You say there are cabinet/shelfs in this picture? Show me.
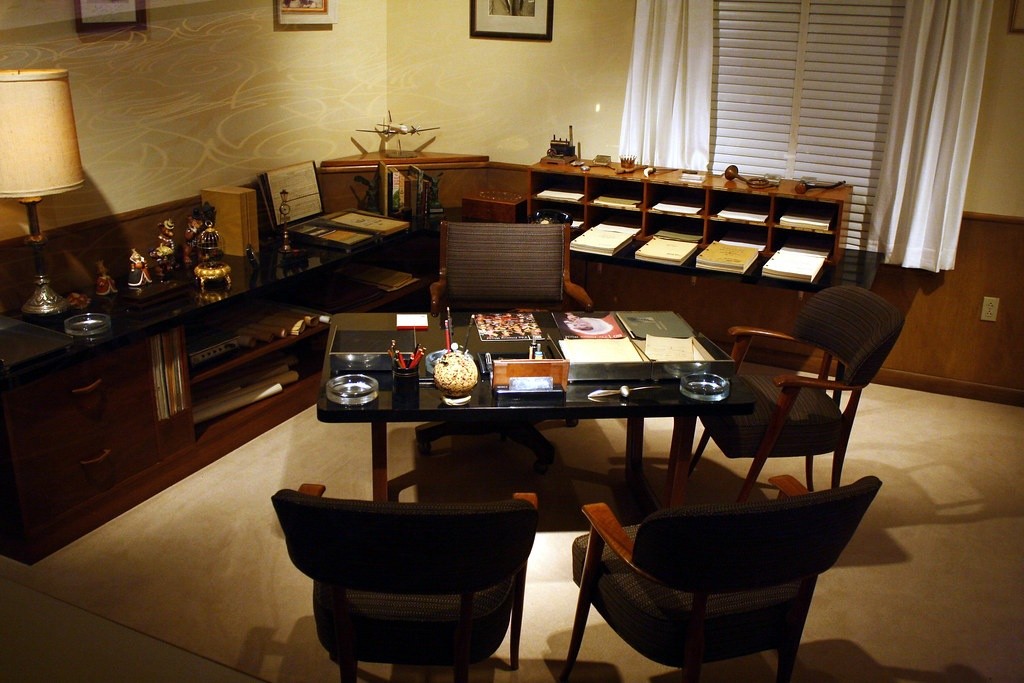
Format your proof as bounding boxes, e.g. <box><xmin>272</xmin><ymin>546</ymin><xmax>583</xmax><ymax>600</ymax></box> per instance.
<box><xmin>0</xmin><ymin>188</ymin><xmax>432</xmax><ymax>567</ymax></box>
<box><xmin>527</xmin><ymin>159</ymin><xmax>855</xmax><ymax>291</ymax></box>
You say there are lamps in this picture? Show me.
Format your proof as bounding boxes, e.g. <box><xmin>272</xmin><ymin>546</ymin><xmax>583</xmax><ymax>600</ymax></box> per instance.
<box><xmin>0</xmin><ymin>68</ymin><xmax>86</xmax><ymax>317</ymax></box>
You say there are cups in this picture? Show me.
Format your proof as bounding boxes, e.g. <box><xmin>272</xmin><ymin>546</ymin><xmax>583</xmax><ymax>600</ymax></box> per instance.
<box><xmin>391</xmin><ymin>358</ymin><xmax>420</xmax><ymax>405</ymax></box>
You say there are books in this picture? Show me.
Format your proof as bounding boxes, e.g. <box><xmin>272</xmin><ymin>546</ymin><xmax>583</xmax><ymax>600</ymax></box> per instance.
<box><xmin>388</xmin><ymin>165</ymin><xmax>430</xmax><ymax>215</ymax></box>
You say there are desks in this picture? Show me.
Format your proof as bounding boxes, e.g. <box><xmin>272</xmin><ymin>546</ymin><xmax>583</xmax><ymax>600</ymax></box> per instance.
<box><xmin>319</xmin><ymin>311</ymin><xmax>758</xmax><ymax>512</ymax></box>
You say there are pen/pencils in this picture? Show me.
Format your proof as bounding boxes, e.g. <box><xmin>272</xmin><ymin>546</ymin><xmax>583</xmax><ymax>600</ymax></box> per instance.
<box><xmin>463</xmin><ymin>314</ymin><xmax>475</xmax><ymax>355</ymax></box>
<box><xmin>445</xmin><ymin>306</ymin><xmax>453</xmax><ymax>353</ymax></box>
<box><xmin>477</xmin><ymin>353</ymin><xmax>487</xmax><ymax>374</ymax></box>
<box><xmin>387</xmin><ymin>338</ymin><xmax>427</xmax><ymax>370</ymax></box>
<box><xmin>531</xmin><ymin>336</ymin><xmax>544</xmax><ymax>360</ymax></box>
<box><xmin>486</xmin><ymin>352</ymin><xmax>492</xmax><ymax>385</ymax></box>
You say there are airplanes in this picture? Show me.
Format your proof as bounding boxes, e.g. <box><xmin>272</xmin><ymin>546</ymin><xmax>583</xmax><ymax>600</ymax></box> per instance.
<box><xmin>355</xmin><ymin>110</ymin><xmax>441</xmax><ymax>136</ymax></box>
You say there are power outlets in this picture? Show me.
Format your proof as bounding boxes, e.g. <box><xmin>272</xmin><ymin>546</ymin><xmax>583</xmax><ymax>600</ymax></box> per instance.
<box><xmin>980</xmin><ymin>297</ymin><xmax>999</xmax><ymax>322</ymax></box>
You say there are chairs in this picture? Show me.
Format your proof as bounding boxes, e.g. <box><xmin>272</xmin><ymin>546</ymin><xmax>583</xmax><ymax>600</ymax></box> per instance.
<box><xmin>270</xmin><ymin>220</ymin><xmax>906</xmax><ymax>682</ymax></box>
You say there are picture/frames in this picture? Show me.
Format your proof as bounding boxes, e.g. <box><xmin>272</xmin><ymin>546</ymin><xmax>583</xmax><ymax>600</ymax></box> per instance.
<box><xmin>469</xmin><ymin>0</ymin><xmax>554</xmax><ymax>41</ymax></box>
<box><xmin>75</xmin><ymin>0</ymin><xmax>147</xmax><ymax>33</ymax></box>
<box><xmin>276</xmin><ymin>0</ymin><xmax>339</xmax><ymax>25</ymax></box>
<box><xmin>1008</xmin><ymin>0</ymin><xmax>1024</xmax><ymax>35</ymax></box>
